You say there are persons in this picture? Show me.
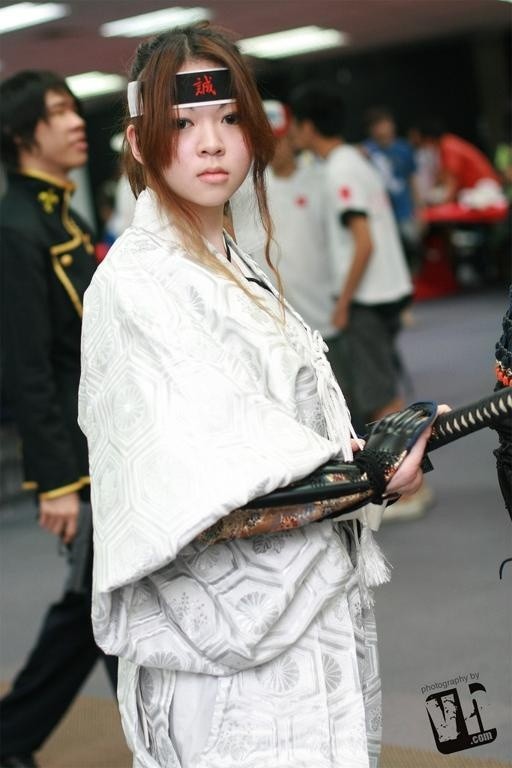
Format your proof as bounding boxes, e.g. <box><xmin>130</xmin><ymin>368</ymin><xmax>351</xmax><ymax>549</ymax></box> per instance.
<box><xmin>77</xmin><ymin>27</ymin><xmax>450</xmax><ymax>768</ymax></box>
<box><xmin>94</xmin><ymin>94</ymin><xmax>512</xmax><ymax>578</ymax></box>
<box><xmin>1</xmin><ymin>71</ymin><xmax>109</xmax><ymax>768</ymax></box>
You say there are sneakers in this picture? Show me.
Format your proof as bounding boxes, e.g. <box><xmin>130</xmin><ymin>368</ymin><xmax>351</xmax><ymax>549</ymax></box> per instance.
<box><xmin>378</xmin><ymin>479</ymin><xmax>437</xmax><ymax>521</ymax></box>
